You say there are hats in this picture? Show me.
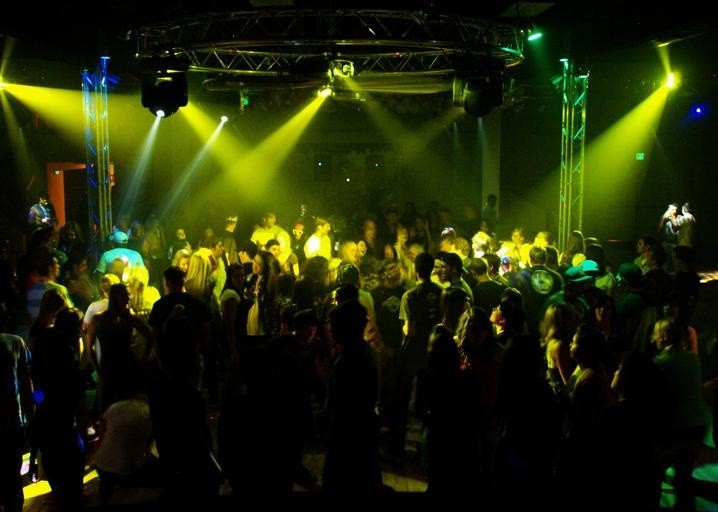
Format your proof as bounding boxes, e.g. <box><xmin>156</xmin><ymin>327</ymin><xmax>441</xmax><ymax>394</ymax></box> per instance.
<box><xmin>236</xmin><ymin>240</ymin><xmax>256</xmax><ymax>257</ymax></box>
<box><xmin>579</xmin><ymin>259</ymin><xmax>599</xmax><ymax>273</ymax></box>
<box><xmin>561</xmin><ymin>266</ymin><xmax>594</xmax><ymax>283</ymax></box>
<box><xmin>440</xmin><ymin>252</ymin><xmax>469</xmax><ymax>276</ymax></box>
<box><xmin>107</xmin><ymin>231</ymin><xmax>129</xmax><ymax>244</ymax></box>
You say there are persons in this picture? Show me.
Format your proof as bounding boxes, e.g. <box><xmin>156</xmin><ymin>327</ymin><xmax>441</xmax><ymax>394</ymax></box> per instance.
<box><xmin>2</xmin><ymin>193</ymin><xmax>717</xmax><ymax>511</ymax></box>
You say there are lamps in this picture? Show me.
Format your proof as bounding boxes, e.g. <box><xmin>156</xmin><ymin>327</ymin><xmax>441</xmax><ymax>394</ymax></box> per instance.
<box><xmin>286</xmin><ymin>56</ymin><xmax>369</xmax><ymax>106</ymax></box>
<box><xmin>137</xmin><ymin>39</ymin><xmax>187</xmax><ymax>121</ymax></box>
<box><xmin>451</xmin><ymin>54</ymin><xmax>505</xmax><ymax>120</ymax></box>
